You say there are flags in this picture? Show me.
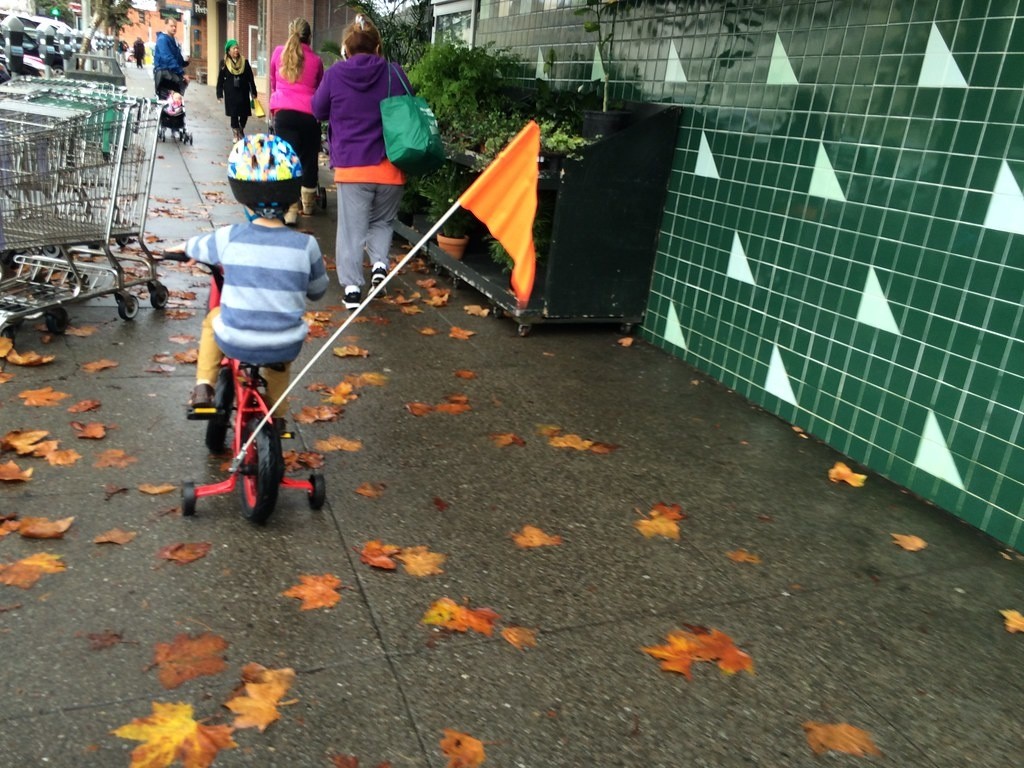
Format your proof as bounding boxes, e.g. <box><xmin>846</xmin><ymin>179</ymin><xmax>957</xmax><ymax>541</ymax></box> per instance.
<box><xmin>459</xmin><ymin>122</ymin><xmax>539</xmax><ymax>307</ymax></box>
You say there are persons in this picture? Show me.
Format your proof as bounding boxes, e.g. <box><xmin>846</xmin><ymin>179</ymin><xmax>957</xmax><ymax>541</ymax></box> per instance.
<box><xmin>162</xmin><ymin>133</ymin><xmax>328</xmax><ymax>433</ymax></box>
<box><xmin>313</xmin><ymin>14</ymin><xmax>414</xmax><ymax>311</ymax></box>
<box><xmin>216</xmin><ymin>39</ymin><xmax>257</xmax><ymax>144</ymax></box>
<box><xmin>268</xmin><ymin>18</ymin><xmax>324</xmax><ymax>225</ymax></box>
<box><xmin>153</xmin><ymin>18</ymin><xmax>191</xmax><ymax>131</ymax></box>
<box><xmin>133</xmin><ymin>36</ymin><xmax>145</xmax><ymax>68</ymax></box>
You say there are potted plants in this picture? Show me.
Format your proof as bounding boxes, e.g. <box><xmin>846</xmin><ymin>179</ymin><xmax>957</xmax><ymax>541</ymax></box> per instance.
<box><xmin>398</xmin><ymin>0</ymin><xmax>642</xmax><ymax>290</ymax></box>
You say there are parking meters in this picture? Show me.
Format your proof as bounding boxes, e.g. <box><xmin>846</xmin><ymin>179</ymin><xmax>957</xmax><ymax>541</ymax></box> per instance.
<box><xmin>0</xmin><ymin>17</ymin><xmax>114</xmax><ymax>73</ymax></box>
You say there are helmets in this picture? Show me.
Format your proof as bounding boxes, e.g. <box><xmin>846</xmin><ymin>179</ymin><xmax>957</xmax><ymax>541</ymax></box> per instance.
<box><xmin>227</xmin><ymin>133</ymin><xmax>303</xmax><ymax>221</ymax></box>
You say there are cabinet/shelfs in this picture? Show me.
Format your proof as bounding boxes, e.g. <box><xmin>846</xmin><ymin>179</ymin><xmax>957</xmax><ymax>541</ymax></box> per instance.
<box><xmin>394</xmin><ymin>91</ymin><xmax>684</xmax><ymax>338</ymax></box>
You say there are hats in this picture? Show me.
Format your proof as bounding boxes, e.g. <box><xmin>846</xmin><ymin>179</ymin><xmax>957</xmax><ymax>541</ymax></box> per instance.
<box><xmin>224</xmin><ymin>39</ymin><xmax>238</xmax><ymax>54</ymax></box>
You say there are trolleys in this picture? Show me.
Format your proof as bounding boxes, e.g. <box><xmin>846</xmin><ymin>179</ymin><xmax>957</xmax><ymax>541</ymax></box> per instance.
<box><xmin>0</xmin><ymin>73</ymin><xmax>172</xmax><ymax>334</ymax></box>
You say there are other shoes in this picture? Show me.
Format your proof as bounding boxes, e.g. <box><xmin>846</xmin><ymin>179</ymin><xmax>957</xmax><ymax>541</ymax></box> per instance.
<box><xmin>232</xmin><ymin>128</ymin><xmax>245</xmax><ymax>141</ymax></box>
<box><xmin>185</xmin><ymin>382</ymin><xmax>218</xmax><ymax>421</ymax></box>
<box><xmin>257</xmin><ymin>416</ymin><xmax>295</xmax><ymax>441</ymax></box>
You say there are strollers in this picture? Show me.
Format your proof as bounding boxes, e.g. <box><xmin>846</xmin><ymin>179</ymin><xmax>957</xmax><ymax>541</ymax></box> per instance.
<box><xmin>155</xmin><ymin>70</ymin><xmax>193</xmax><ymax>145</ymax></box>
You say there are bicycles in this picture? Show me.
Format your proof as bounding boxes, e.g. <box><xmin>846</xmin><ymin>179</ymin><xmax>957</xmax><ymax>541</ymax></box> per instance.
<box><xmin>162</xmin><ymin>250</ymin><xmax>326</xmax><ymax>526</ymax></box>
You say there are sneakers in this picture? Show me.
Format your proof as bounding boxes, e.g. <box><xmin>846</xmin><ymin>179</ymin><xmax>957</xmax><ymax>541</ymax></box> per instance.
<box><xmin>342</xmin><ymin>290</ymin><xmax>361</xmax><ymax>310</ymax></box>
<box><xmin>368</xmin><ymin>266</ymin><xmax>388</xmax><ymax>301</ymax></box>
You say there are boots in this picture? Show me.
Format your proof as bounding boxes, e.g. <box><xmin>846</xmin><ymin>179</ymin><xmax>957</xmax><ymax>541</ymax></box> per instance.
<box><xmin>284</xmin><ymin>202</ymin><xmax>299</xmax><ymax>224</ymax></box>
<box><xmin>301</xmin><ymin>186</ymin><xmax>319</xmax><ymax>218</ymax></box>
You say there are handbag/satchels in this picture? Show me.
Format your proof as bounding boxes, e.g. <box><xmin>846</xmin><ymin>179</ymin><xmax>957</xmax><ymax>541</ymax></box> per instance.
<box><xmin>250</xmin><ymin>97</ymin><xmax>266</xmax><ymax>118</ymax></box>
<box><xmin>379</xmin><ymin>61</ymin><xmax>443</xmax><ymax>163</ymax></box>
